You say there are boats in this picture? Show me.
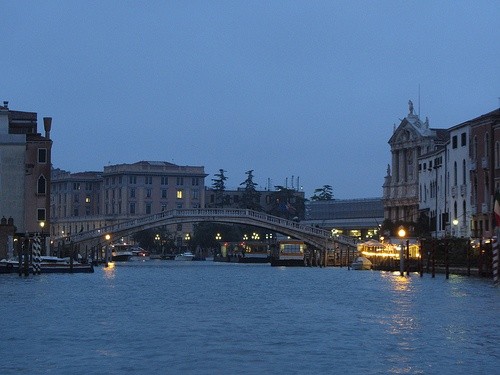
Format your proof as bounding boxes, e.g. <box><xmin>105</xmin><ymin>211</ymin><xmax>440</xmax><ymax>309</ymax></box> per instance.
<box><xmin>95</xmin><ymin>233</ymin><xmax>319</xmax><ymax>267</ymax></box>
<box><xmin>0</xmin><ymin>254</ymin><xmax>94</xmax><ymax>274</ymax></box>
<box><xmin>353</xmin><ymin>254</ymin><xmax>373</xmax><ymax>270</ymax></box>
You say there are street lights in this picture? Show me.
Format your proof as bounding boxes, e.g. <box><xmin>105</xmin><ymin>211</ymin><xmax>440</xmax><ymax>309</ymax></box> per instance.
<box><xmin>398</xmin><ymin>228</ymin><xmax>407</xmax><ymax>277</ymax></box>
<box><xmin>41</xmin><ymin>221</ymin><xmax>44</xmax><ymax>255</ymax></box>
